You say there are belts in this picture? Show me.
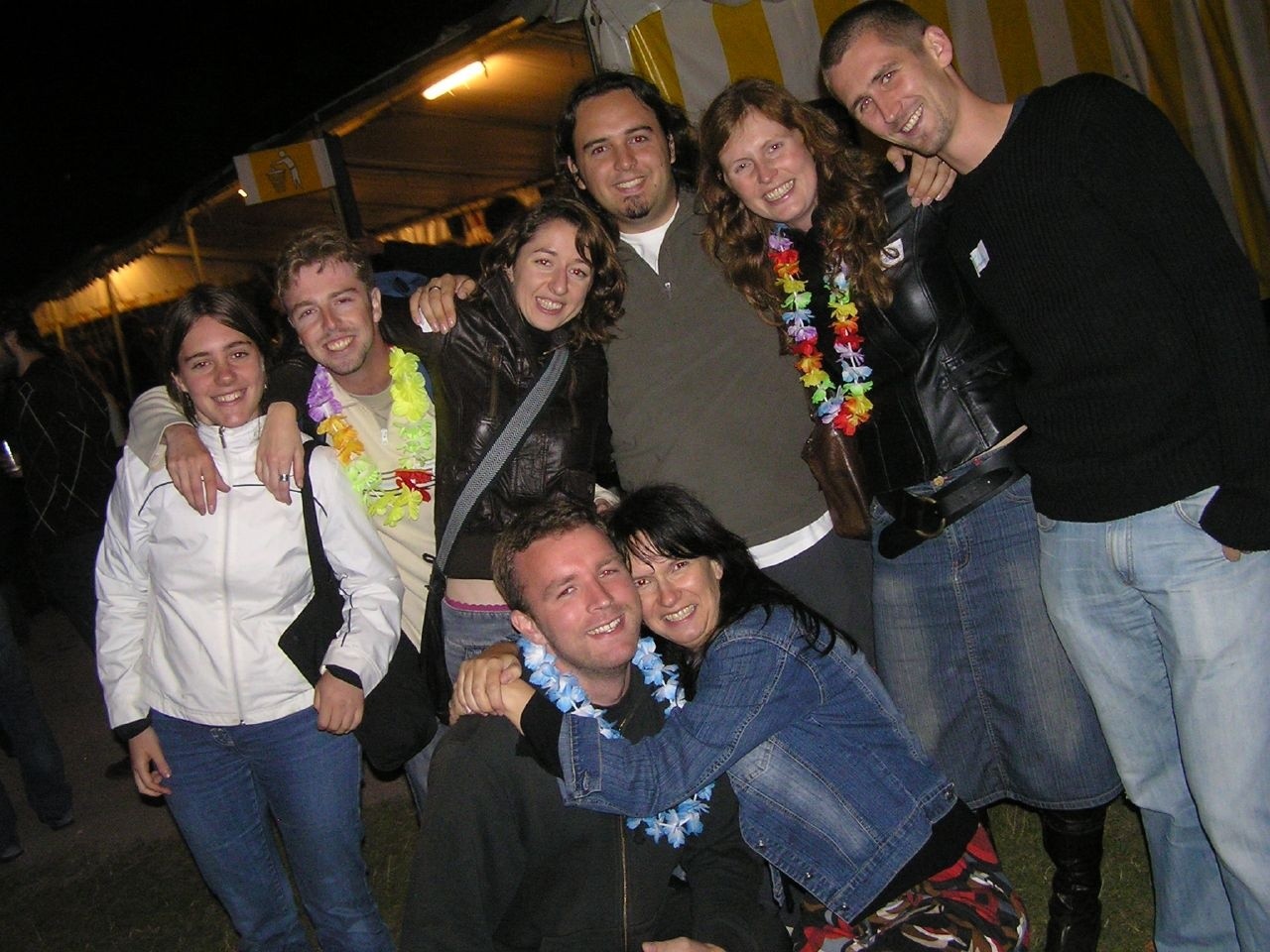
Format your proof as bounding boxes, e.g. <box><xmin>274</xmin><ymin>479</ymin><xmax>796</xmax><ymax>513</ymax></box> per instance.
<box><xmin>875</xmin><ymin>429</ymin><xmax>1033</xmax><ymax>560</ymax></box>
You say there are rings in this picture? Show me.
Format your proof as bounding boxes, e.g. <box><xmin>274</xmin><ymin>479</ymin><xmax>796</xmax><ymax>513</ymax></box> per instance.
<box><xmin>938</xmin><ymin>160</ymin><xmax>946</xmax><ymax>164</ymax></box>
<box><xmin>428</xmin><ymin>286</ymin><xmax>441</xmax><ymax>292</ymax></box>
<box><xmin>279</xmin><ymin>474</ymin><xmax>288</xmax><ymax>481</ymax></box>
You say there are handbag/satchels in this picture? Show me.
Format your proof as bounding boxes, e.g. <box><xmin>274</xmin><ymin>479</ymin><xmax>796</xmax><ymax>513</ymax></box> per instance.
<box><xmin>278</xmin><ymin>583</ymin><xmax>438</xmax><ymax>772</ymax></box>
<box><xmin>421</xmin><ymin>554</ymin><xmax>454</xmax><ymax>726</ymax></box>
<box><xmin>802</xmin><ymin>413</ymin><xmax>872</xmax><ymax>540</ymax></box>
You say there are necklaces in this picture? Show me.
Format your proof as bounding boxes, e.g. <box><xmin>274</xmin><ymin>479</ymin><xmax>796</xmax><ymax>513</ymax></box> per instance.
<box><xmin>305</xmin><ymin>347</ymin><xmax>436</xmax><ymax>532</ymax></box>
<box><xmin>515</xmin><ymin>635</ymin><xmax>716</xmax><ymax>848</ymax></box>
<box><xmin>765</xmin><ymin>227</ymin><xmax>874</xmax><ymax>437</ymax></box>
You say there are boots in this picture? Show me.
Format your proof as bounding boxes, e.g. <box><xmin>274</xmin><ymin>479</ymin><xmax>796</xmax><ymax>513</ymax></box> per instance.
<box><xmin>1039</xmin><ymin>809</ymin><xmax>1105</xmax><ymax>952</ymax></box>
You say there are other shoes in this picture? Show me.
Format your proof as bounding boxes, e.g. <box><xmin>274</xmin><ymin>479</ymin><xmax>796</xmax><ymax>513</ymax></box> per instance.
<box><xmin>50</xmin><ymin>808</ymin><xmax>73</xmax><ymax>831</ymax></box>
<box><xmin>0</xmin><ymin>844</ymin><xmax>24</xmax><ymax>862</ymax></box>
<box><xmin>105</xmin><ymin>756</ymin><xmax>134</xmax><ymax>777</ymax></box>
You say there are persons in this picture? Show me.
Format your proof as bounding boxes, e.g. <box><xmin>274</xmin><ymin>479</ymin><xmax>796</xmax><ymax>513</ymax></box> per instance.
<box><xmin>409</xmin><ymin>49</ymin><xmax>956</xmax><ymax>683</ymax></box>
<box><xmin>692</xmin><ymin>72</ymin><xmax>1120</xmax><ymax>952</ymax></box>
<box><xmin>816</xmin><ymin>0</ymin><xmax>1270</xmax><ymax>952</ymax></box>
<box><xmin>1</xmin><ymin>302</ymin><xmax>127</xmax><ymax>860</ymax></box>
<box><xmin>256</xmin><ymin>196</ymin><xmax>628</xmax><ymax>825</ymax></box>
<box><xmin>449</xmin><ymin>484</ymin><xmax>1032</xmax><ymax>952</ymax></box>
<box><xmin>397</xmin><ymin>487</ymin><xmax>812</xmax><ymax>952</ymax></box>
<box><xmin>92</xmin><ymin>230</ymin><xmax>446</xmax><ymax>952</ymax></box>
